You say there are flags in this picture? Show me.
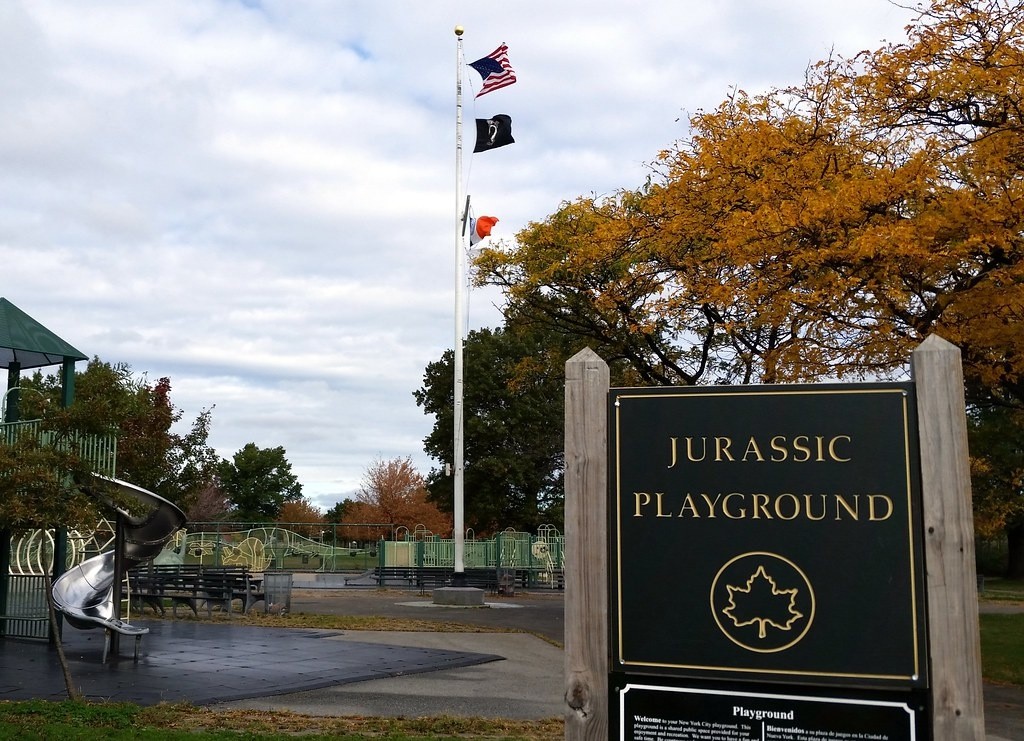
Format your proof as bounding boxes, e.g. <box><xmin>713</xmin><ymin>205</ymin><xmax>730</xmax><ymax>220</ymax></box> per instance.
<box><xmin>473</xmin><ymin>114</ymin><xmax>515</xmax><ymax>153</ymax></box>
<box><xmin>470</xmin><ymin>216</ymin><xmax>500</xmax><ymax>246</ymax></box>
<box><xmin>467</xmin><ymin>42</ymin><xmax>516</xmax><ymax>98</ymax></box>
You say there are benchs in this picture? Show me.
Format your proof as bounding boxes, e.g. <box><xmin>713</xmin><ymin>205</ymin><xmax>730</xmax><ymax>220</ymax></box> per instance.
<box><xmin>0</xmin><ymin>575</ymin><xmax>52</xmax><ymax>639</ymax></box>
<box><xmin>371</xmin><ymin>567</ymin><xmax>529</xmax><ymax>597</ymax></box>
<box><xmin>121</xmin><ymin>564</ymin><xmax>266</xmax><ymax>621</ymax></box>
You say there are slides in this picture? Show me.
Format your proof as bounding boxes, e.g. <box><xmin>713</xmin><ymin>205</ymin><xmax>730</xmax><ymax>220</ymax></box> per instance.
<box><xmin>48</xmin><ymin>463</ymin><xmax>189</xmax><ymax>635</ymax></box>
